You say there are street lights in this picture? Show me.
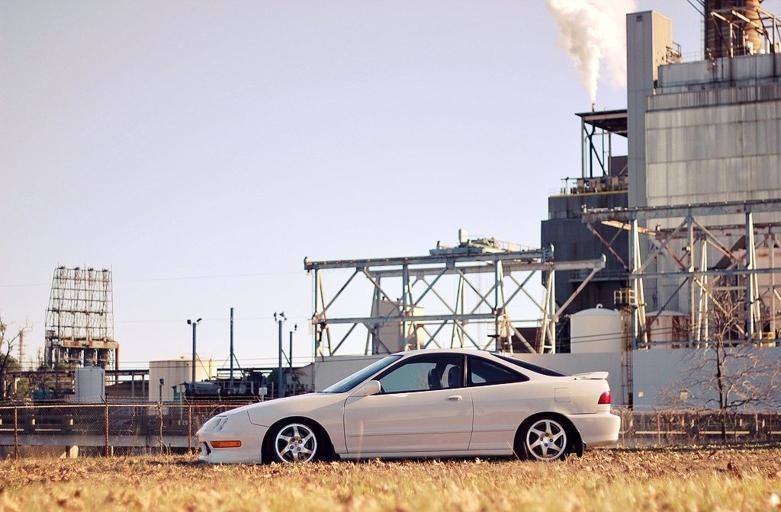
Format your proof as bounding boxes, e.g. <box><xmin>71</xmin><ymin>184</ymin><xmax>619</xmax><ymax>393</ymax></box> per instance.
<box><xmin>159</xmin><ymin>377</ymin><xmax>165</xmax><ymax>404</ymax></box>
<box><xmin>273</xmin><ymin>312</ymin><xmax>287</xmax><ymax>397</ymax></box>
<box><xmin>290</xmin><ymin>323</ymin><xmax>299</xmax><ymax>391</ymax></box>
<box><xmin>186</xmin><ymin>318</ymin><xmax>204</xmax><ymax>401</ymax></box>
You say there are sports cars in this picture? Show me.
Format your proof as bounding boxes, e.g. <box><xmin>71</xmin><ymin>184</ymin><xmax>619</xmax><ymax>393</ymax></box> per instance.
<box><xmin>194</xmin><ymin>349</ymin><xmax>621</xmax><ymax>468</ymax></box>
